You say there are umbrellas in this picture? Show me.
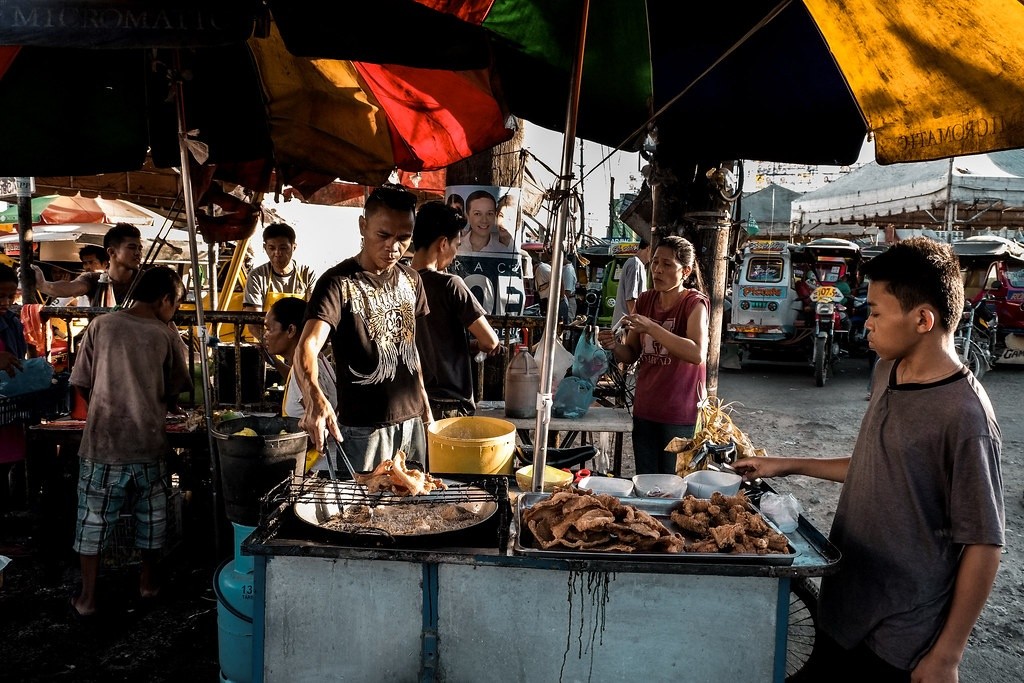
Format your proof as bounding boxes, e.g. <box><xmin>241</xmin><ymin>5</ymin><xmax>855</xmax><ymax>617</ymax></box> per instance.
<box><xmin>419</xmin><ymin>0</ymin><xmax>1024</xmax><ymax>510</ymax></box>
<box><xmin>0</xmin><ymin>0</ymin><xmax>517</xmax><ymax>522</ymax></box>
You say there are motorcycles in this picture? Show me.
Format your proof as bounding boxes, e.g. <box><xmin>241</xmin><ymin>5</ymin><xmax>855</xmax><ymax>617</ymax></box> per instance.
<box><xmin>595</xmin><ymin>254</ymin><xmax>636</xmax><ymax>330</ymax></box>
<box><xmin>798</xmin><ymin>238</ymin><xmax>866</xmax><ymax>385</ymax></box>
<box><xmin>950</xmin><ymin>235</ymin><xmax>1024</xmax><ymax>380</ymax></box>
<box><xmin>729</xmin><ymin>240</ymin><xmax>803</xmax><ymax>349</ymax></box>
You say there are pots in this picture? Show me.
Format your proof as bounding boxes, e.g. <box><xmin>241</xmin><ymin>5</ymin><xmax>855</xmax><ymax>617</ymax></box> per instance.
<box><xmin>293</xmin><ymin>478</ymin><xmax>499</xmax><ymax>545</ymax></box>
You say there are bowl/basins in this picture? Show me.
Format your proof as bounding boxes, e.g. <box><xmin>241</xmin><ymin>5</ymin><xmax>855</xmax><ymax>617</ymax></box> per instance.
<box><xmin>515</xmin><ymin>463</ymin><xmax>574</xmax><ymax>492</ymax></box>
<box><xmin>632</xmin><ymin>474</ymin><xmax>687</xmax><ymax>498</ymax></box>
<box><xmin>684</xmin><ymin>469</ymin><xmax>742</xmax><ymax>498</ymax></box>
<box><xmin>578</xmin><ymin>476</ymin><xmax>633</xmax><ymax>497</ymax></box>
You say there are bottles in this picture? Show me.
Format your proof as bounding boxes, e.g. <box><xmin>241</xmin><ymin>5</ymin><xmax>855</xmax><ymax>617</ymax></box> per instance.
<box><xmin>504</xmin><ymin>346</ymin><xmax>539</xmax><ymax>417</ymax></box>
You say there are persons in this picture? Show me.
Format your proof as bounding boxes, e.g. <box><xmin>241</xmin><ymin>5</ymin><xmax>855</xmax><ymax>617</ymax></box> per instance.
<box><xmin>292</xmin><ymin>182</ymin><xmax>438</xmax><ymax>475</ymax></box>
<box><xmin>262</xmin><ymin>296</ymin><xmax>339</xmax><ymax>480</ymax></box>
<box><xmin>241</xmin><ymin>222</ymin><xmax>322</xmax><ymax>397</ymax></box>
<box><xmin>491</xmin><ymin>195</ymin><xmax>521</xmax><ymax>253</ymax></box>
<box><xmin>727</xmin><ymin>236</ymin><xmax>1007</xmax><ymax>683</ymax></box>
<box><xmin>0</xmin><ymin>221</ymin><xmax>194</xmax><ymax>618</ymax></box>
<box><xmin>406</xmin><ymin>199</ymin><xmax>500</xmax><ymax>421</ymax></box>
<box><xmin>608</xmin><ymin>238</ymin><xmax>650</xmax><ymax>390</ymax></box>
<box><xmin>534</xmin><ymin>247</ymin><xmax>578</xmax><ymax>330</ymax></box>
<box><xmin>445</xmin><ymin>193</ymin><xmax>465</xmax><ymax>216</ymax></box>
<box><xmin>458</xmin><ymin>190</ymin><xmax>507</xmax><ymax>252</ymax></box>
<box><xmin>596</xmin><ymin>234</ymin><xmax>711</xmax><ymax>476</ymax></box>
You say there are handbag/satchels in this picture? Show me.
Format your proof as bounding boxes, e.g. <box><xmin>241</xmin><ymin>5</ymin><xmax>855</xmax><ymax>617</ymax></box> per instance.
<box><xmin>551</xmin><ymin>375</ymin><xmax>594</xmax><ymax>418</ymax></box>
<box><xmin>572</xmin><ymin>330</ymin><xmax>610</xmax><ymax>380</ymax></box>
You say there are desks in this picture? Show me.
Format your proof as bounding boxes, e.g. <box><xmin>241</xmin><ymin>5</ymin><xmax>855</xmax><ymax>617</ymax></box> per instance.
<box><xmin>28</xmin><ymin>405</ymin><xmax>635</xmax><ymax>481</ymax></box>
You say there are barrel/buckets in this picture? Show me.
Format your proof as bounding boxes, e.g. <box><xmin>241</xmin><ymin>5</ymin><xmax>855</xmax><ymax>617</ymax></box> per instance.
<box><xmin>427</xmin><ymin>416</ymin><xmax>516</xmax><ymax>474</ymax></box>
<box><xmin>211</xmin><ymin>416</ymin><xmax>309</xmax><ymax>525</ymax></box>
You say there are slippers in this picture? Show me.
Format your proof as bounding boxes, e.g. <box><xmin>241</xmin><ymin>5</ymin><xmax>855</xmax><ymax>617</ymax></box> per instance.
<box><xmin>59</xmin><ymin>595</ymin><xmax>95</xmax><ymax>621</ymax></box>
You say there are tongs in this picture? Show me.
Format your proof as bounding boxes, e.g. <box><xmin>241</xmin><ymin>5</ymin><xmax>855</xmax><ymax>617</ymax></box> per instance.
<box><xmin>323</xmin><ymin>427</ymin><xmax>368</xmax><ymax>515</ymax></box>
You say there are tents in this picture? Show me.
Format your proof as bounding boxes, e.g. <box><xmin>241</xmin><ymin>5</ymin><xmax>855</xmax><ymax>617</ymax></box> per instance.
<box><xmin>727</xmin><ymin>145</ymin><xmax>1024</xmax><ymax>237</ymax></box>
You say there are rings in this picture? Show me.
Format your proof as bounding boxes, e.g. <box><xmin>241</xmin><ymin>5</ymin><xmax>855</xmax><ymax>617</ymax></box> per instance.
<box><xmin>600</xmin><ymin>340</ymin><xmax>603</xmax><ymax>344</ymax></box>
